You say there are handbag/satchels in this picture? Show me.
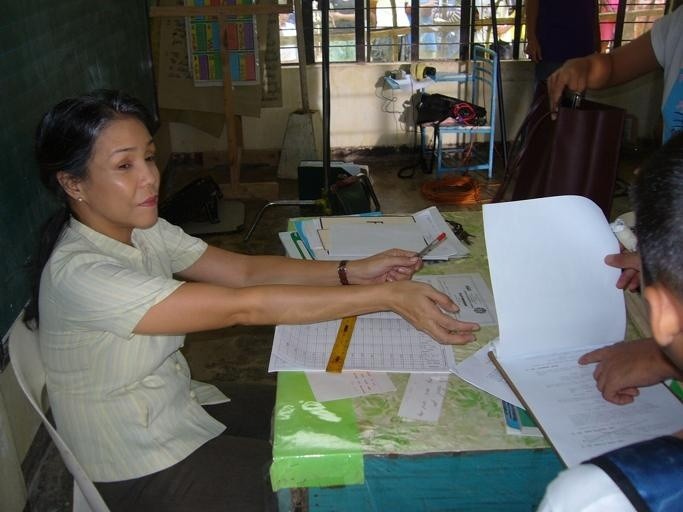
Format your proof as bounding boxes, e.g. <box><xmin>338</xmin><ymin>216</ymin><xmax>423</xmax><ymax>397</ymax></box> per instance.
<box><xmin>492</xmin><ymin>80</ymin><xmax>626</xmax><ymax>221</ymax></box>
<box><xmin>412</xmin><ymin>91</ymin><xmax>486</xmax><ymax>126</ymax></box>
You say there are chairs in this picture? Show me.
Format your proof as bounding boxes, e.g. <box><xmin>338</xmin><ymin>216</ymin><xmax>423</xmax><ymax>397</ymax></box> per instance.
<box><xmin>6</xmin><ymin>303</ymin><xmax>111</xmax><ymax>511</ymax></box>
<box><xmin>419</xmin><ymin>44</ymin><xmax>499</xmax><ymax>181</ymax></box>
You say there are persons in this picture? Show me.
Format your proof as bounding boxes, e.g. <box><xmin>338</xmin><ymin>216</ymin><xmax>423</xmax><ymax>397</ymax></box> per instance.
<box><xmin>524</xmin><ymin>1</ymin><xmax>600</xmax><ymax>106</ymax></box>
<box><xmin>537</xmin><ymin>126</ymin><xmax>683</xmax><ymax>512</ymax></box>
<box><xmin>22</xmin><ymin>88</ymin><xmax>482</xmax><ymax>512</ymax></box>
<box><xmin>547</xmin><ymin>5</ymin><xmax>683</xmax><ymax>146</ymax></box>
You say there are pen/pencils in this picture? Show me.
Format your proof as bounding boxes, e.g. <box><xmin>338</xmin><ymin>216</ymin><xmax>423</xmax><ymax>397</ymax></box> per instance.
<box><xmin>416</xmin><ymin>233</ymin><xmax>445</xmax><ymax>257</ymax></box>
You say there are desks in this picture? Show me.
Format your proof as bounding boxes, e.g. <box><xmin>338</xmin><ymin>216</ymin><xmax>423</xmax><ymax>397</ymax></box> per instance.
<box><xmin>262</xmin><ymin>206</ymin><xmax>567</xmax><ymax>511</ymax></box>
<box><xmin>383</xmin><ymin>72</ymin><xmax>476</xmax><ymax>97</ymax></box>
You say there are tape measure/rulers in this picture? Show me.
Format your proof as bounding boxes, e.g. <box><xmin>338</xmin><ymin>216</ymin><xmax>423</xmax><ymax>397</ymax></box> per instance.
<box><xmin>326</xmin><ymin>317</ymin><xmax>356</xmax><ymax>372</ymax></box>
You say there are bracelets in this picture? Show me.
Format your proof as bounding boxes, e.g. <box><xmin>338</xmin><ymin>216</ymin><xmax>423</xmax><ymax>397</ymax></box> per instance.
<box><xmin>336</xmin><ymin>258</ymin><xmax>351</xmax><ymax>287</ymax></box>
